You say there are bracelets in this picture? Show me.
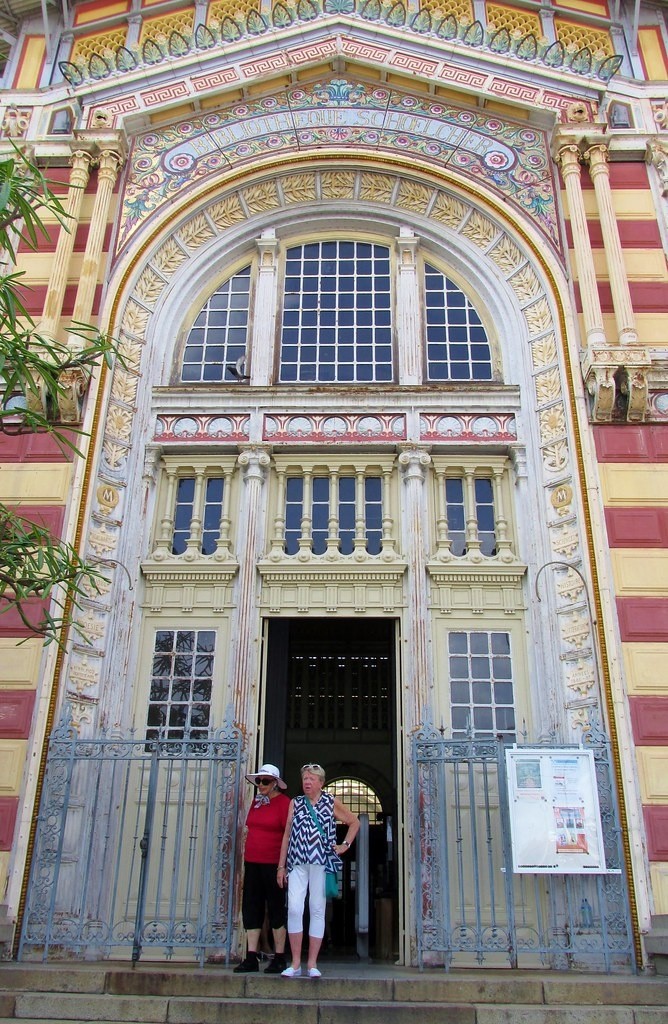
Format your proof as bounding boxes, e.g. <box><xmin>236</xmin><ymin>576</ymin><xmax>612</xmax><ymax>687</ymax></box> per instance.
<box><xmin>341</xmin><ymin>840</ymin><xmax>352</xmax><ymax>849</ymax></box>
<box><xmin>276</xmin><ymin>865</ymin><xmax>285</xmax><ymax>871</ymax></box>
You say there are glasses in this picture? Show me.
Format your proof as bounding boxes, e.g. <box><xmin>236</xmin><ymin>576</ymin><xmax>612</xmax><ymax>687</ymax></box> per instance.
<box><xmin>303</xmin><ymin>765</ymin><xmax>322</xmax><ymax>770</ymax></box>
<box><xmin>255</xmin><ymin>777</ymin><xmax>275</xmax><ymax>786</ymax></box>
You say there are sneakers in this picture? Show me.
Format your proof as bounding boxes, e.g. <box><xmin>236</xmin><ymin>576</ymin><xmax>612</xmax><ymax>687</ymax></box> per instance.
<box><xmin>307</xmin><ymin>968</ymin><xmax>321</xmax><ymax>977</ymax></box>
<box><xmin>264</xmin><ymin>958</ymin><xmax>286</xmax><ymax>973</ymax></box>
<box><xmin>281</xmin><ymin>966</ymin><xmax>301</xmax><ymax>976</ymax></box>
<box><xmin>234</xmin><ymin>957</ymin><xmax>259</xmax><ymax>972</ymax></box>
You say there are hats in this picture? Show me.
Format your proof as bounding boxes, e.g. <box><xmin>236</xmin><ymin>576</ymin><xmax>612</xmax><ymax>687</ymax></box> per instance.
<box><xmin>245</xmin><ymin>764</ymin><xmax>287</xmax><ymax>789</ymax></box>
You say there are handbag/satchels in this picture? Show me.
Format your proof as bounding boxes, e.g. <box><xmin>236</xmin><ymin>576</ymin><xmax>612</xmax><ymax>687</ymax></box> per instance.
<box><xmin>326</xmin><ymin>872</ymin><xmax>339</xmax><ymax>897</ymax></box>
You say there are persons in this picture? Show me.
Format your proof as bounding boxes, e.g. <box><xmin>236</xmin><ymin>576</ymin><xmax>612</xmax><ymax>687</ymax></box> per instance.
<box><xmin>233</xmin><ymin>764</ymin><xmax>291</xmax><ymax>973</ymax></box>
<box><xmin>276</xmin><ymin>765</ymin><xmax>360</xmax><ymax>977</ymax></box>
